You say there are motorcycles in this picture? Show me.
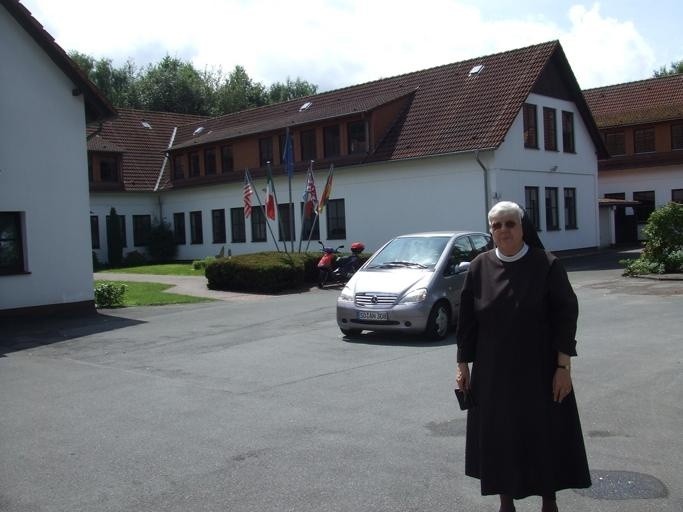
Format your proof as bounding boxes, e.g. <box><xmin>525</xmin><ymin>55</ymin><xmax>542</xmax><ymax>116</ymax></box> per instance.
<box><xmin>316</xmin><ymin>240</ymin><xmax>363</xmax><ymax>288</ymax></box>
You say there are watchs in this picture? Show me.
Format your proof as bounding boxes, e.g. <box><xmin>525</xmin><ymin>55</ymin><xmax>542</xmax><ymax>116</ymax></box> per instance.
<box><xmin>556</xmin><ymin>364</ymin><xmax>570</xmax><ymax>371</ymax></box>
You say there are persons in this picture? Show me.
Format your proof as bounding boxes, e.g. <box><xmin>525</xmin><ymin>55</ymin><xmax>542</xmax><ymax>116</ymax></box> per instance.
<box><xmin>454</xmin><ymin>201</ymin><xmax>591</xmax><ymax>512</ymax></box>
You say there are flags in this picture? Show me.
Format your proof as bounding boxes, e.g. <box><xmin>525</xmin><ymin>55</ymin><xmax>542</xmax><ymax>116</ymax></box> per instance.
<box><xmin>241</xmin><ymin>128</ymin><xmax>335</xmax><ymax>222</ymax></box>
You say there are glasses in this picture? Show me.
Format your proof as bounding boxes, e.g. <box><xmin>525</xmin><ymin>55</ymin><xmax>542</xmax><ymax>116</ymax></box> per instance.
<box><xmin>492</xmin><ymin>220</ymin><xmax>516</xmax><ymax>230</ymax></box>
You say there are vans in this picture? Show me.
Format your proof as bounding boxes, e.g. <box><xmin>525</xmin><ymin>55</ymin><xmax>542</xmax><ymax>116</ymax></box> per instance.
<box><xmin>337</xmin><ymin>232</ymin><xmax>491</xmax><ymax>342</ymax></box>
